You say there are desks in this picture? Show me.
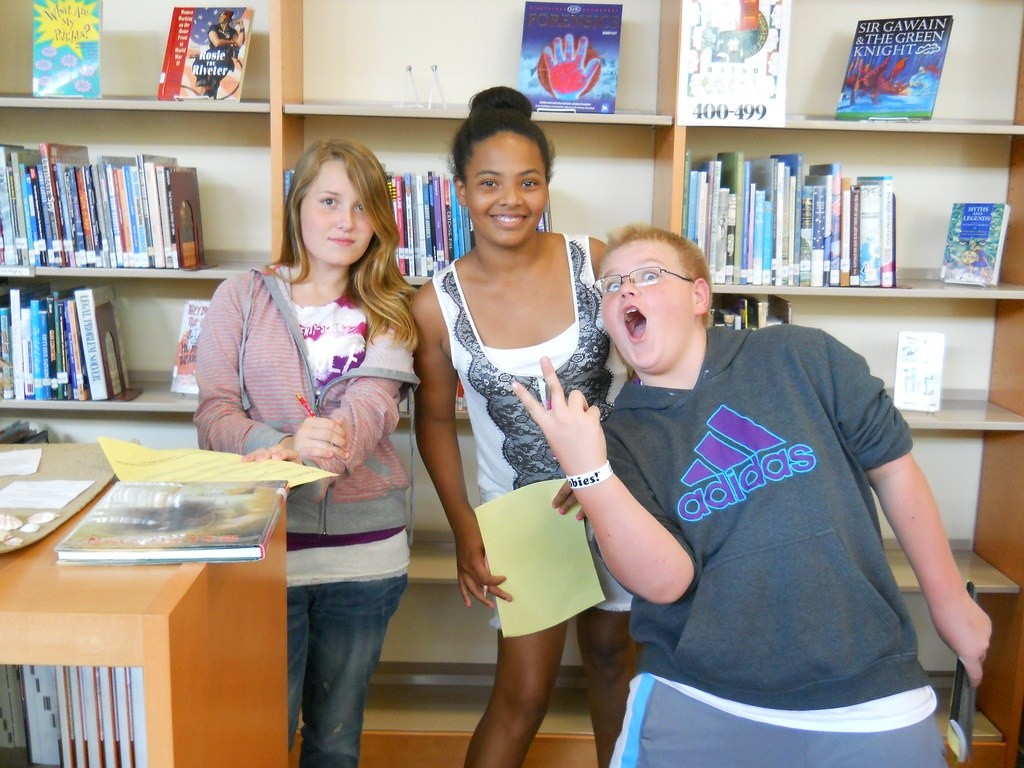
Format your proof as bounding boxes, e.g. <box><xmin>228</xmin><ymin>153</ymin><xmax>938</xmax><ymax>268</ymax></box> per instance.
<box><xmin>0</xmin><ymin>483</ymin><xmax>290</xmax><ymax>768</ymax></box>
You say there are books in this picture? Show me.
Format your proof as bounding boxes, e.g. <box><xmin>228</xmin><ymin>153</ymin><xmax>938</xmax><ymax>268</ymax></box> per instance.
<box><xmin>284</xmin><ymin>170</ymin><xmax>296</xmax><ymax>199</ymax></box>
<box><xmin>383</xmin><ymin>172</ymin><xmax>476</xmax><ymax>278</ymax></box>
<box><xmin>941</xmin><ymin>203</ymin><xmax>1011</xmax><ymax>286</ymax></box>
<box><xmin>535</xmin><ymin>193</ymin><xmax>552</xmax><ymax>233</ymax></box>
<box><xmin>710</xmin><ymin>295</ymin><xmax>792</xmax><ymax>331</ymax></box>
<box><xmin>0</xmin><ymin>142</ymin><xmax>204</xmax><ymax>269</ymax></box>
<box><xmin>680</xmin><ymin>148</ymin><xmax>897</xmax><ymax>289</ymax></box>
<box><xmin>0</xmin><ymin>283</ymin><xmax>130</xmax><ymax>401</ymax></box>
<box><xmin>170</xmin><ymin>299</ymin><xmax>211</xmax><ymax>395</ymax></box>
<box><xmin>56</xmin><ymin>479</ymin><xmax>288</xmax><ymax>563</ymax></box>
<box><xmin>834</xmin><ymin>15</ymin><xmax>954</xmax><ymax>119</ymax></box>
<box><xmin>32</xmin><ymin>0</ymin><xmax>103</xmax><ymax>99</ymax></box>
<box><xmin>932</xmin><ymin>687</ymin><xmax>1002</xmax><ymax>742</ymax></box>
<box><xmin>158</xmin><ymin>6</ymin><xmax>254</xmax><ymax>102</ymax></box>
<box><xmin>518</xmin><ymin>1</ymin><xmax>623</xmax><ymax>114</ymax></box>
<box><xmin>893</xmin><ymin>331</ymin><xmax>945</xmax><ymax>413</ymax></box>
<box><xmin>0</xmin><ymin>420</ymin><xmax>49</xmax><ymax>444</ymax></box>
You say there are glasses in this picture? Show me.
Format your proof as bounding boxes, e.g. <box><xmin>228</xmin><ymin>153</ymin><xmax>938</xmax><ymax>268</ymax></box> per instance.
<box><xmin>593</xmin><ymin>265</ymin><xmax>694</xmax><ymax>297</ymax></box>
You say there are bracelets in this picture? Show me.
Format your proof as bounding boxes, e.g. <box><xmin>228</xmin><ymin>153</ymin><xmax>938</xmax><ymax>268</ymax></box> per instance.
<box><xmin>567</xmin><ymin>461</ymin><xmax>613</xmax><ymax>489</ymax></box>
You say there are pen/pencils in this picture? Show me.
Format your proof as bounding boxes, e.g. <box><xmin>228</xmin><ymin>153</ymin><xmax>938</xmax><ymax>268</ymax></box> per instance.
<box><xmin>294</xmin><ymin>392</ymin><xmax>316</xmax><ymax>419</ymax></box>
<box><xmin>482</xmin><ymin>552</ymin><xmax>489</xmax><ymax>599</ymax></box>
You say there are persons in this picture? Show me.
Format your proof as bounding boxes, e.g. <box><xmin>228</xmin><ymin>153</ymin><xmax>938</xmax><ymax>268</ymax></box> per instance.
<box><xmin>408</xmin><ymin>88</ymin><xmax>639</xmax><ymax>768</ymax></box>
<box><xmin>513</xmin><ymin>225</ymin><xmax>993</xmax><ymax>768</ymax></box>
<box><xmin>193</xmin><ymin>138</ymin><xmax>421</xmax><ymax>768</ymax></box>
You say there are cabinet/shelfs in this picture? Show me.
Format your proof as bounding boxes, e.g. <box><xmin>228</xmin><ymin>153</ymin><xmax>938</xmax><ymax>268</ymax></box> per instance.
<box><xmin>1</xmin><ymin>0</ymin><xmax>1022</xmax><ymax>767</ymax></box>
